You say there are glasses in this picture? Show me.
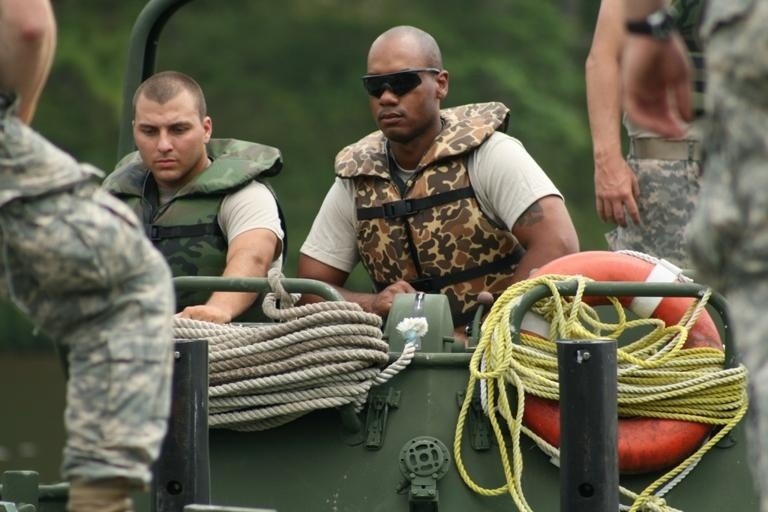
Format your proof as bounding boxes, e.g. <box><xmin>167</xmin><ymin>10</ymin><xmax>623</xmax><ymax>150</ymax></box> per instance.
<box><xmin>360</xmin><ymin>68</ymin><xmax>440</xmax><ymax>99</ymax></box>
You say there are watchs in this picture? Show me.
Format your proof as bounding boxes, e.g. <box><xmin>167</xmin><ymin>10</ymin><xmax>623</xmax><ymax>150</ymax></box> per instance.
<box><xmin>626</xmin><ymin>10</ymin><xmax>676</xmax><ymax>39</ymax></box>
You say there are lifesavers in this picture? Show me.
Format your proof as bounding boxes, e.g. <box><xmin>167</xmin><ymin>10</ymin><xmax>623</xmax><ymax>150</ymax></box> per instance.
<box><xmin>495</xmin><ymin>250</ymin><xmax>723</xmax><ymax>471</ymax></box>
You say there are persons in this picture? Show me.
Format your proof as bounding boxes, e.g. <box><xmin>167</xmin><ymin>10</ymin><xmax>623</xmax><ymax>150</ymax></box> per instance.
<box><xmin>586</xmin><ymin>0</ymin><xmax>708</xmax><ymax>271</ymax></box>
<box><xmin>101</xmin><ymin>71</ymin><xmax>287</xmax><ymax>325</ymax></box>
<box><xmin>294</xmin><ymin>25</ymin><xmax>580</xmax><ymax>347</ymax></box>
<box><xmin>0</xmin><ymin>0</ymin><xmax>176</xmax><ymax>512</ymax></box>
<box><xmin>621</xmin><ymin>0</ymin><xmax>768</xmax><ymax>512</ymax></box>
